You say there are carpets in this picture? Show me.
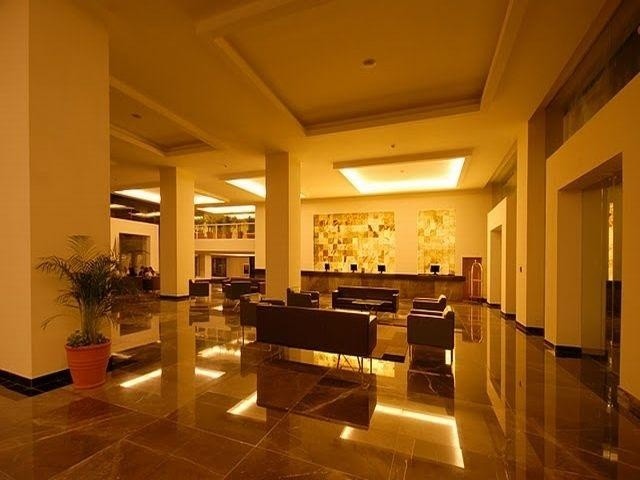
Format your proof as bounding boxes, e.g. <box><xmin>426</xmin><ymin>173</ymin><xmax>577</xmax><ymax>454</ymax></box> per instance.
<box><xmin>366</xmin><ymin>322</ymin><xmax>409</xmax><ymax>364</ymax></box>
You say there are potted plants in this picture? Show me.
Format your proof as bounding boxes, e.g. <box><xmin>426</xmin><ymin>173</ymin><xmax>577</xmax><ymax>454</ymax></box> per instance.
<box><xmin>32</xmin><ymin>232</ymin><xmax>142</xmax><ymax>389</ymax></box>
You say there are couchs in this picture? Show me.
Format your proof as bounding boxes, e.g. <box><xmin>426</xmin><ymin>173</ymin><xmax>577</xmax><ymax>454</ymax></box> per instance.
<box><xmin>331</xmin><ymin>286</ymin><xmax>399</xmax><ymax>319</ymax></box>
<box><xmin>256</xmin><ymin>302</ymin><xmax>377</xmax><ymax>376</ymax></box>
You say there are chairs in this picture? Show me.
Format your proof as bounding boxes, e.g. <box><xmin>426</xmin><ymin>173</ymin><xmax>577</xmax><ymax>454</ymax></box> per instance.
<box><xmin>287</xmin><ymin>288</ymin><xmax>320</xmax><ymax>308</ymax></box>
<box><xmin>124</xmin><ymin>274</ymin><xmax>285</xmax><ymax>339</ymax></box>
<box><xmin>406</xmin><ymin>304</ymin><xmax>455</xmax><ymax>364</ymax></box>
<box><xmin>412</xmin><ymin>293</ymin><xmax>447</xmax><ymax>311</ymax></box>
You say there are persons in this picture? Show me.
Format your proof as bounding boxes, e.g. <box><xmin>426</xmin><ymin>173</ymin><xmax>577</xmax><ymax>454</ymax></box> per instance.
<box><xmin>127</xmin><ymin>266</ymin><xmax>156</xmax><ymax>280</ymax></box>
